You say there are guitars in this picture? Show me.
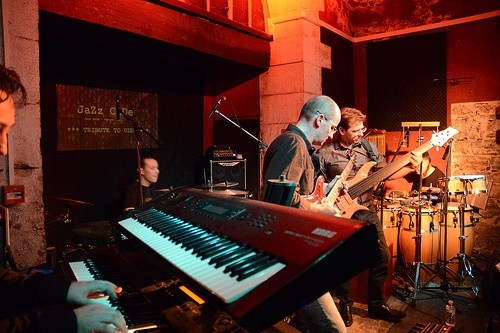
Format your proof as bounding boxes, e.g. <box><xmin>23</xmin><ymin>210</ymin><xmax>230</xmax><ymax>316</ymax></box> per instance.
<box><xmin>334</xmin><ymin>125</ymin><xmax>462</xmax><ymax>219</ymax></box>
<box><xmin>300</xmin><ymin>151</ymin><xmax>356</xmax><ymax>206</ymax></box>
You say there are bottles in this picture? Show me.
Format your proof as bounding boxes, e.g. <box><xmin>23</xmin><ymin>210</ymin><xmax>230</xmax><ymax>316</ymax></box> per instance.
<box><xmin>445</xmin><ymin>301</ymin><xmax>456</xmax><ymax>327</ymax></box>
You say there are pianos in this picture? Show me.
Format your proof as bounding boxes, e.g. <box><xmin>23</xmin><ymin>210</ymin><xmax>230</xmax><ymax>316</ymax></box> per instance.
<box><xmin>56</xmin><ymin>186</ymin><xmax>382</xmax><ymax>333</ymax></box>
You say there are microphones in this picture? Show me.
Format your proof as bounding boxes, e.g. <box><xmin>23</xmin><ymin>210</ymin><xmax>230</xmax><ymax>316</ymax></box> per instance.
<box><xmin>208</xmin><ymin>99</ymin><xmax>222</xmax><ymax>119</ymax></box>
<box><xmin>407</xmin><ymin>127</ymin><xmax>409</xmax><ymax>147</ymax></box>
<box><xmin>116</xmin><ymin>95</ymin><xmax>120</xmax><ymax>120</ymax></box>
<box><xmin>442</xmin><ymin>144</ymin><xmax>449</xmax><ymax>160</ymax></box>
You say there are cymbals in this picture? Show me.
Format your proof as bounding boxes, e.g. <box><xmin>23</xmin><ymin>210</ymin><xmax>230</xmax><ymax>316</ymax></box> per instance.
<box><xmin>156</xmin><ymin>189</ymin><xmax>248</xmax><ymax>196</ymax></box>
<box><xmin>214</xmin><ymin>182</ymin><xmax>239</xmax><ymax>187</ymax></box>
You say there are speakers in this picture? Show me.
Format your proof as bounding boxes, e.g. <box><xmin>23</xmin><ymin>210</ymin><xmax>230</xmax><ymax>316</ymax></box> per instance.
<box><xmin>205</xmin><ymin>158</ymin><xmax>247</xmax><ymax>198</ymax></box>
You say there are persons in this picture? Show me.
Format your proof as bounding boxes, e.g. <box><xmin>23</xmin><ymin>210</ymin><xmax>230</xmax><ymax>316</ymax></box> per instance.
<box><xmin>317</xmin><ymin>108</ymin><xmax>422</xmax><ymax>327</ymax></box>
<box><xmin>262</xmin><ymin>94</ymin><xmax>350</xmax><ymax>333</ymax></box>
<box><xmin>124</xmin><ymin>155</ymin><xmax>159</xmax><ymax>211</ymax></box>
<box><xmin>405</xmin><ymin>150</ymin><xmax>445</xmax><ymax>193</ymax></box>
<box><xmin>0</xmin><ymin>65</ymin><xmax>127</xmax><ymax>333</ymax></box>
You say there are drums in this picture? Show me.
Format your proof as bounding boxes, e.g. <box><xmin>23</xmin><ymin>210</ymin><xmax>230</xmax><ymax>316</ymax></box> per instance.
<box><xmin>373</xmin><ymin>202</ymin><xmax>401</xmax><ymax>272</ymax></box>
<box><xmin>399</xmin><ymin>206</ymin><xmax>440</xmax><ymax>285</ymax></box>
<box><xmin>436</xmin><ymin>202</ymin><xmax>474</xmax><ymax>282</ymax></box>
<box><xmin>439</xmin><ymin>174</ymin><xmax>487</xmax><ymax>196</ymax></box>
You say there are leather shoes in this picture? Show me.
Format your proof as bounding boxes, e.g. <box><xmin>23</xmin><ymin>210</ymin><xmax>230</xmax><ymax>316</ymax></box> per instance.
<box><xmin>368</xmin><ymin>305</ymin><xmax>405</xmax><ymax>321</ymax></box>
<box><xmin>340</xmin><ymin>305</ymin><xmax>353</xmax><ymax>325</ymax></box>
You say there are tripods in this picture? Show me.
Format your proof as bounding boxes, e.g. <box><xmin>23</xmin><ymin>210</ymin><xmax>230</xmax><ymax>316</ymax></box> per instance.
<box><xmin>392</xmin><ymin>127</ymin><xmax>484</xmax><ymax>308</ymax></box>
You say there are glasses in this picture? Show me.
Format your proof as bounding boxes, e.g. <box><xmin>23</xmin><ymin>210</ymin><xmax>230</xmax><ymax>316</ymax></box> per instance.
<box><xmin>317</xmin><ymin>110</ymin><xmax>338</xmax><ymax>135</ymax></box>
<box><xmin>345</xmin><ymin>128</ymin><xmax>367</xmax><ymax>135</ymax></box>
